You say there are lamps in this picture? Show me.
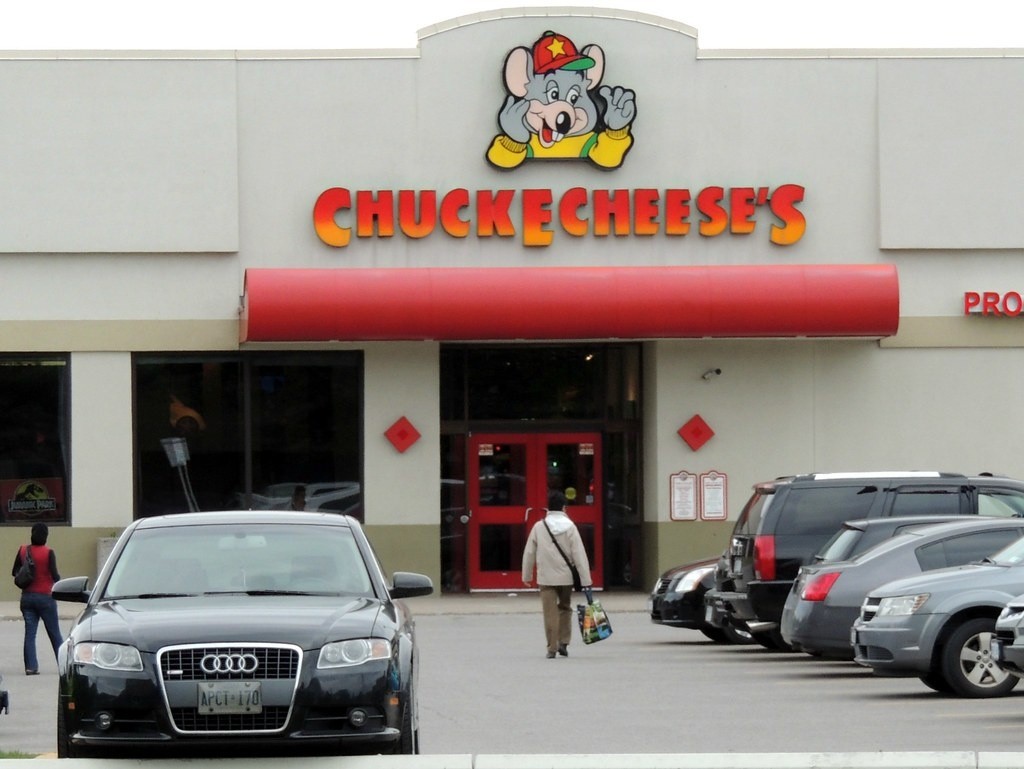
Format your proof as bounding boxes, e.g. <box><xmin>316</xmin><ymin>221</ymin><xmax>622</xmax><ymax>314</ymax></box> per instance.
<box><xmin>702</xmin><ymin>368</ymin><xmax>721</xmax><ymax>380</ymax></box>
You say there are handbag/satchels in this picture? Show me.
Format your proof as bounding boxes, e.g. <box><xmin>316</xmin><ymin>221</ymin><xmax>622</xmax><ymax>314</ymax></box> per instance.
<box><xmin>572</xmin><ymin>566</ymin><xmax>582</xmax><ymax>593</ymax></box>
<box><xmin>14</xmin><ymin>546</ymin><xmax>35</xmax><ymax>590</ymax></box>
<box><xmin>576</xmin><ymin>586</ymin><xmax>612</xmax><ymax>644</ymax></box>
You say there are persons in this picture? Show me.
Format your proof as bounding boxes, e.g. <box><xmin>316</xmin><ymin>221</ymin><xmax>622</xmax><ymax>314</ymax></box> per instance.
<box><xmin>12</xmin><ymin>523</ymin><xmax>63</xmax><ymax>675</ymax></box>
<box><xmin>522</xmin><ymin>495</ymin><xmax>592</xmax><ymax>658</ymax></box>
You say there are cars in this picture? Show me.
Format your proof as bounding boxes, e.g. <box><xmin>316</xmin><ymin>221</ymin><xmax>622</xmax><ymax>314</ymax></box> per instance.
<box><xmin>227</xmin><ymin>482</ymin><xmax>641</xmax><ymax>587</ymax></box>
<box><xmin>780</xmin><ymin>515</ymin><xmax>1024</xmax><ymax>698</ymax></box>
<box><xmin>52</xmin><ymin>507</ymin><xmax>433</xmax><ymax>759</ymax></box>
<box><xmin>652</xmin><ymin>550</ymin><xmax>727</xmax><ymax>642</ymax></box>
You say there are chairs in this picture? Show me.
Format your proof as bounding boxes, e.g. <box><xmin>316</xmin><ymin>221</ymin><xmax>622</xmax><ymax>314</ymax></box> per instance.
<box><xmin>160</xmin><ymin>559</ymin><xmax>203</xmax><ymax>587</ymax></box>
<box><xmin>291</xmin><ymin>556</ymin><xmax>334</xmax><ymax>581</ymax></box>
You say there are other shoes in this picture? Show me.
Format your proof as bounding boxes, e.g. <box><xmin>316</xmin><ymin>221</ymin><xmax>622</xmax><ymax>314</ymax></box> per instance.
<box><xmin>546</xmin><ymin>651</ymin><xmax>556</xmax><ymax>658</ymax></box>
<box><xmin>558</xmin><ymin>644</ymin><xmax>568</xmax><ymax>657</ymax></box>
<box><xmin>26</xmin><ymin>668</ymin><xmax>40</xmax><ymax>676</ymax></box>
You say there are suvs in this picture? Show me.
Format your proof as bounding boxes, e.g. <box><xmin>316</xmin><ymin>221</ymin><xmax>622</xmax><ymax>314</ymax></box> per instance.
<box><xmin>700</xmin><ymin>469</ymin><xmax>1024</xmax><ymax>649</ymax></box>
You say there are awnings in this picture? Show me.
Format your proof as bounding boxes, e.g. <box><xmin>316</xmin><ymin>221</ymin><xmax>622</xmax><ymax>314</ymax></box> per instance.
<box><xmin>238</xmin><ymin>264</ymin><xmax>899</xmax><ymax>339</ymax></box>
